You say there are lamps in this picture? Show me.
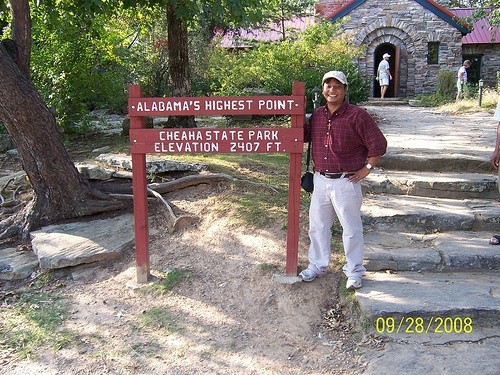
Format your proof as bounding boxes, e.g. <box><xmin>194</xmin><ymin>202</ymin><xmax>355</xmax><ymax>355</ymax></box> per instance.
<box><xmin>311</xmin><ymin>86</ymin><xmax>321</xmax><ymax>102</ymax></box>
<box><xmin>479</xmin><ymin>78</ymin><xmax>484</xmax><ymax>86</ymax></box>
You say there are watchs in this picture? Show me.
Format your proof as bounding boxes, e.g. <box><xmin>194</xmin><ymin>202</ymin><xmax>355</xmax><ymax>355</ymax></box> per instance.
<box><xmin>365</xmin><ymin>164</ymin><xmax>373</xmax><ymax>169</ymax></box>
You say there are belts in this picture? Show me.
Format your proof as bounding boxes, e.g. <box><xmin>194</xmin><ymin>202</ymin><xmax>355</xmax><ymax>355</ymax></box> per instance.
<box><xmin>320</xmin><ymin>172</ymin><xmax>354</xmax><ymax>179</ymax></box>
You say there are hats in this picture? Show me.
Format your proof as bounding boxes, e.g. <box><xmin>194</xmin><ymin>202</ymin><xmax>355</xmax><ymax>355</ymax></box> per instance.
<box><xmin>383</xmin><ymin>53</ymin><xmax>391</xmax><ymax>59</ymax></box>
<box><xmin>322</xmin><ymin>71</ymin><xmax>348</xmax><ymax>91</ymax></box>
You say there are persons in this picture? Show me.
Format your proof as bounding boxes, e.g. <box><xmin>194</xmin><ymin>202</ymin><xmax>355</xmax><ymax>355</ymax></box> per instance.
<box><xmin>456</xmin><ymin>60</ymin><xmax>471</xmax><ymax>99</ymax></box>
<box><xmin>489</xmin><ymin>102</ymin><xmax>500</xmax><ymax>244</ymax></box>
<box><xmin>298</xmin><ymin>71</ymin><xmax>388</xmax><ymax>290</ymax></box>
<box><xmin>376</xmin><ymin>53</ymin><xmax>392</xmax><ymax>98</ymax></box>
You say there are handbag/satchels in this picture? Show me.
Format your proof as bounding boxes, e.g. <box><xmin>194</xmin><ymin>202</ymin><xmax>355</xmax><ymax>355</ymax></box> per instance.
<box><xmin>300</xmin><ymin>172</ymin><xmax>313</xmax><ymax>193</ymax></box>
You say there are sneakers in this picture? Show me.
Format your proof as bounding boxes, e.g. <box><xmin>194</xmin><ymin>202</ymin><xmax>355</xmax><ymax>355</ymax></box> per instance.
<box><xmin>346</xmin><ymin>276</ymin><xmax>362</xmax><ymax>290</ymax></box>
<box><xmin>298</xmin><ymin>268</ymin><xmax>327</xmax><ymax>282</ymax></box>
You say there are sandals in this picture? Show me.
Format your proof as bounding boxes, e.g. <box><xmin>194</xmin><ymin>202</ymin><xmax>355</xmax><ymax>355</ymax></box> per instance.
<box><xmin>489</xmin><ymin>234</ymin><xmax>500</xmax><ymax>245</ymax></box>
